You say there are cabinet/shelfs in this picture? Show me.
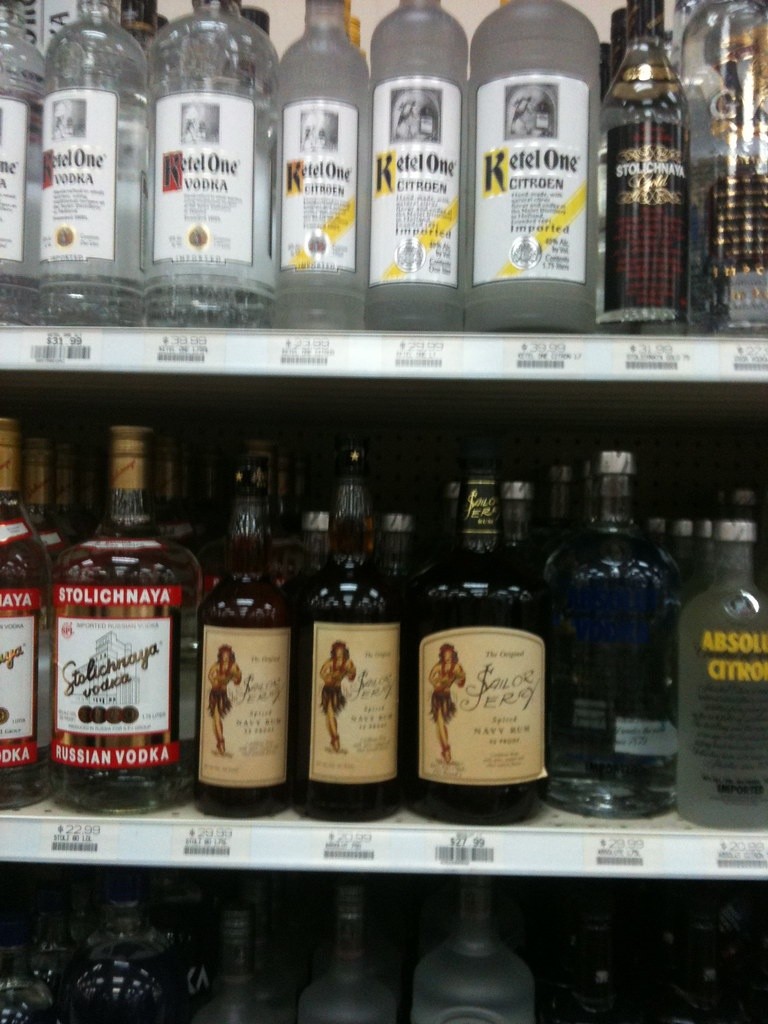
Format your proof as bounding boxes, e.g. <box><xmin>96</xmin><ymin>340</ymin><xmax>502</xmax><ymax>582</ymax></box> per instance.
<box><xmin>0</xmin><ymin>322</ymin><xmax>768</xmax><ymax>875</ymax></box>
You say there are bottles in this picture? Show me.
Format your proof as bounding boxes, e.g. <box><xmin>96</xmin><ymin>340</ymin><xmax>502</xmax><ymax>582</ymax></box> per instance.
<box><xmin>1</xmin><ymin>863</ymin><xmax>768</xmax><ymax>1024</ymax></box>
<box><xmin>0</xmin><ymin>415</ymin><xmax>768</xmax><ymax>828</ymax></box>
<box><xmin>0</xmin><ymin>0</ymin><xmax>768</xmax><ymax>339</ymax></box>
